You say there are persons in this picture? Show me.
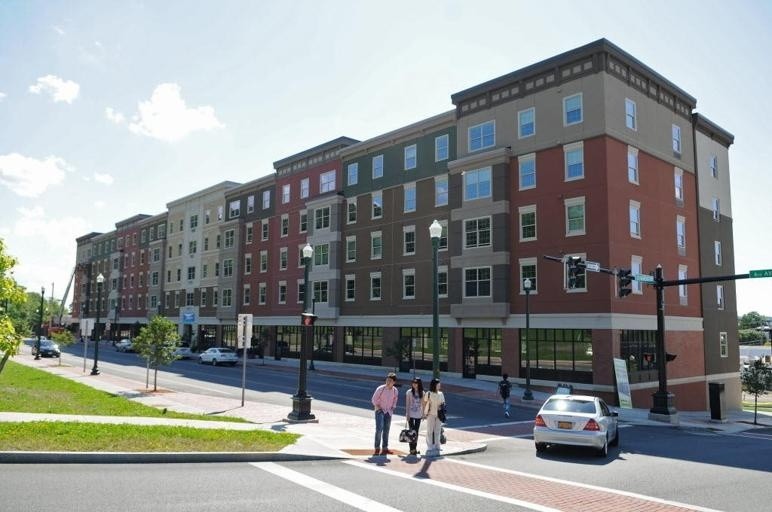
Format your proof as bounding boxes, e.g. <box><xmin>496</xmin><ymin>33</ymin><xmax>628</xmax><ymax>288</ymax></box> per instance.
<box><xmin>404</xmin><ymin>377</ymin><xmax>426</xmax><ymax>455</ymax></box>
<box><xmin>421</xmin><ymin>378</ymin><xmax>447</xmax><ymax>453</ymax></box>
<box><xmin>496</xmin><ymin>373</ymin><xmax>513</xmax><ymax>419</ymax></box>
<box><xmin>371</xmin><ymin>371</ymin><xmax>399</xmax><ymax>456</ymax></box>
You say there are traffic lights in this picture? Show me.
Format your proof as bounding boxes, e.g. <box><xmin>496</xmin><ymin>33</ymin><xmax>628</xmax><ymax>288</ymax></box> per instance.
<box><xmin>301</xmin><ymin>314</ymin><xmax>316</xmax><ymax>327</ymax></box>
<box><xmin>564</xmin><ymin>254</ymin><xmax>587</xmax><ymax>288</ymax></box>
<box><xmin>667</xmin><ymin>353</ymin><xmax>677</xmax><ymax>362</ymax></box>
<box><xmin>617</xmin><ymin>267</ymin><xmax>635</xmax><ymax>298</ymax></box>
<box><xmin>643</xmin><ymin>354</ymin><xmax>654</xmax><ymax>361</ymax></box>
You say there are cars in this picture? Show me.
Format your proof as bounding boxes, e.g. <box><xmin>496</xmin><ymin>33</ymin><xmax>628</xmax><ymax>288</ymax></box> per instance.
<box><xmin>530</xmin><ymin>391</ymin><xmax>620</xmax><ymax>458</ymax></box>
<box><xmin>198</xmin><ymin>347</ymin><xmax>240</xmax><ymax>367</ymax></box>
<box><xmin>586</xmin><ymin>347</ymin><xmax>593</xmax><ymax>356</ymax></box>
<box><xmin>113</xmin><ymin>338</ymin><xmax>138</xmax><ymax>353</ymax></box>
<box><xmin>31</xmin><ymin>338</ymin><xmax>61</xmax><ymax>358</ymax></box>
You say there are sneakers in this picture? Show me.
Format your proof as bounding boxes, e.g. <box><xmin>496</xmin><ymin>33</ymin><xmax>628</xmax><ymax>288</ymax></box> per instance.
<box><xmin>409</xmin><ymin>448</ymin><xmax>443</xmax><ymax>455</ymax></box>
<box><xmin>374</xmin><ymin>449</ymin><xmax>380</xmax><ymax>455</ymax></box>
<box><xmin>382</xmin><ymin>449</ymin><xmax>394</xmax><ymax>455</ymax></box>
<box><xmin>503</xmin><ymin>412</ymin><xmax>510</xmax><ymax>419</ymax></box>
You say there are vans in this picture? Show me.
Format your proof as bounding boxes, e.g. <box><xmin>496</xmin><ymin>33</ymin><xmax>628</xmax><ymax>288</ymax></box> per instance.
<box><xmin>255</xmin><ymin>341</ymin><xmax>289</xmax><ymax>356</ymax></box>
<box><xmin>172</xmin><ymin>341</ymin><xmax>192</xmax><ymax>359</ymax></box>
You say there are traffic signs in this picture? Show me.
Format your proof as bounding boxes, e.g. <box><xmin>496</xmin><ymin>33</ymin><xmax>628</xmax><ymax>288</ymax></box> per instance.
<box><xmin>749</xmin><ymin>269</ymin><xmax>772</xmax><ymax>278</ymax></box>
<box><xmin>635</xmin><ymin>273</ymin><xmax>654</xmax><ymax>282</ymax></box>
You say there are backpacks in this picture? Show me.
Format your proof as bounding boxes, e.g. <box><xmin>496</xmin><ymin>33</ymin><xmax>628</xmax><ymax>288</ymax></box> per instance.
<box><xmin>499</xmin><ymin>381</ymin><xmax>512</xmax><ymax>398</ymax></box>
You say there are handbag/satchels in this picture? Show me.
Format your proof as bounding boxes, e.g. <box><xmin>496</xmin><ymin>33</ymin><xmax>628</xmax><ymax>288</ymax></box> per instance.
<box><xmin>399</xmin><ymin>429</ymin><xmax>419</xmax><ymax>443</ymax></box>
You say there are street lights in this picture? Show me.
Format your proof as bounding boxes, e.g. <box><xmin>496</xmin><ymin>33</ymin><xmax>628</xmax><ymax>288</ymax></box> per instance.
<box><xmin>34</xmin><ymin>284</ymin><xmax>46</xmax><ymax>360</ymax></box>
<box><xmin>308</xmin><ymin>293</ymin><xmax>318</xmax><ymax>371</ymax></box>
<box><xmin>521</xmin><ymin>277</ymin><xmax>535</xmax><ymax>401</ymax></box>
<box><xmin>112</xmin><ymin>302</ymin><xmax>120</xmax><ymax>340</ymax></box>
<box><xmin>157</xmin><ymin>301</ymin><xmax>162</xmax><ymax>315</ymax></box>
<box><xmin>89</xmin><ymin>272</ymin><xmax>106</xmax><ymax>377</ymax></box>
<box><xmin>280</xmin><ymin>240</ymin><xmax>319</xmax><ymax>424</ymax></box>
<box><xmin>427</xmin><ymin>218</ymin><xmax>444</xmax><ymax>381</ymax></box>
